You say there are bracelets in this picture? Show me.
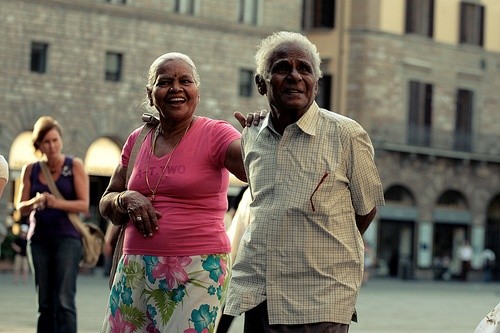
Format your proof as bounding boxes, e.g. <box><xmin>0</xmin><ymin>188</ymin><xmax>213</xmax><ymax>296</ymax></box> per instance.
<box><xmin>119</xmin><ymin>191</ymin><xmax>126</xmax><ymax>212</ymax></box>
<box><xmin>115</xmin><ymin>194</ymin><xmax>124</xmax><ymax>215</ymax></box>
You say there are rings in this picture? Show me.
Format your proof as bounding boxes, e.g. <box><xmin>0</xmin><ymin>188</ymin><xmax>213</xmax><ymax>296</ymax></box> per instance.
<box><xmin>136</xmin><ymin>217</ymin><xmax>141</xmax><ymax>221</ymax></box>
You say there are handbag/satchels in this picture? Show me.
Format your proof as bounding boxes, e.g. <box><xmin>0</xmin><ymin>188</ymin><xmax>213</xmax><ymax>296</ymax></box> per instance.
<box><xmin>78</xmin><ymin>222</ymin><xmax>105</xmax><ymax>269</ymax></box>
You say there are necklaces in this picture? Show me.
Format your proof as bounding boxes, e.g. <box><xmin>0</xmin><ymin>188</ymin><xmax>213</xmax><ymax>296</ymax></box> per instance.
<box><xmin>46</xmin><ymin>161</ymin><xmax>58</xmax><ymax>174</ymax></box>
<box><xmin>145</xmin><ymin>116</ymin><xmax>191</xmax><ymax>200</ymax></box>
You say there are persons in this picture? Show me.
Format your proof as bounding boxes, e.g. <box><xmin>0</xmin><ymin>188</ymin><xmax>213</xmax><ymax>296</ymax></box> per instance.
<box><xmin>434</xmin><ymin>255</ymin><xmax>450</xmax><ymax>279</ymax></box>
<box><xmin>98</xmin><ymin>52</ymin><xmax>267</xmax><ymax>333</ymax></box>
<box><xmin>361</xmin><ymin>241</ymin><xmax>375</xmax><ymax>285</ymax></box>
<box><xmin>17</xmin><ymin>116</ymin><xmax>89</xmax><ymax>333</ymax></box>
<box><xmin>0</xmin><ymin>154</ymin><xmax>9</xmax><ymax>200</ymax></box>
<box><xmin>459</xmin><ymin>240</ymin><xmax>473</xmax><ymax>281</ymax></box>
<box><xmin>224</xmin><ymin>31</ymin><xmax>385</xmax><ymax>333</ymax></box>
<box><xmin>1</xmin><ymin>201</ymin><xmax>121</xmax><ymax>274</ymax></box>
